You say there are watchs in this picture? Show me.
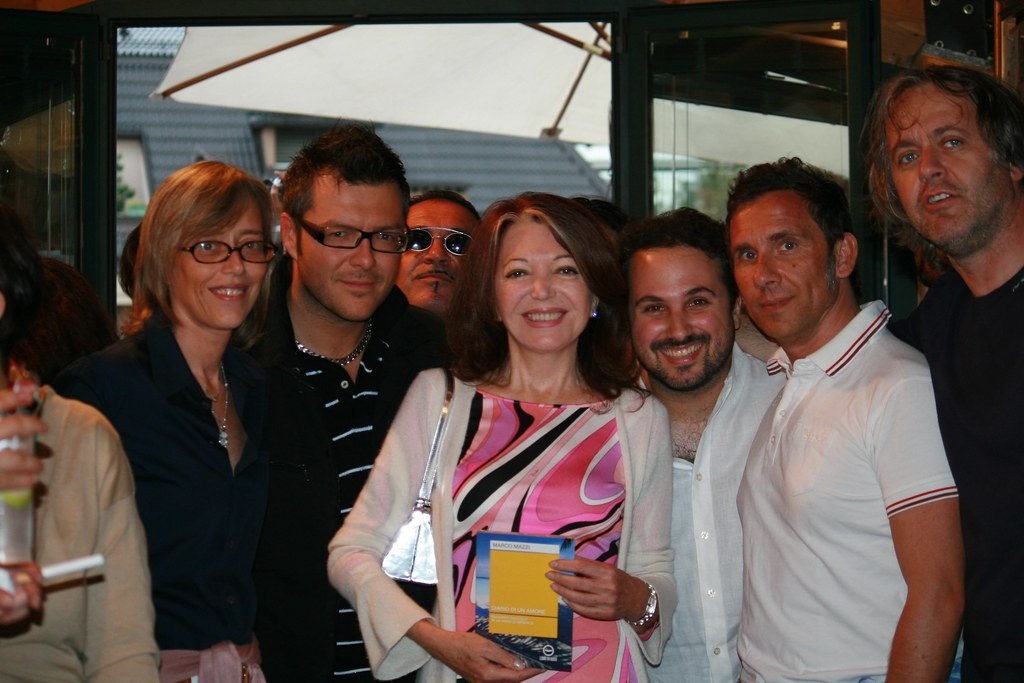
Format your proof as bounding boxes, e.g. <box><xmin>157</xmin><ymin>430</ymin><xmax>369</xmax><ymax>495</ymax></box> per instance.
<box><xmin>624</xmin><ymin>578</ymin><xmax>659</xmax><ymax>626</ymax></box>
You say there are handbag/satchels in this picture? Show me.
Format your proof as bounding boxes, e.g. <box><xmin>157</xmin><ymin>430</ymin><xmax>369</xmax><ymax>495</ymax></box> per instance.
<box><xmin>382</xmin><ymin>367</ymin><xmax>456</xmax><ymax>585</ymax></box>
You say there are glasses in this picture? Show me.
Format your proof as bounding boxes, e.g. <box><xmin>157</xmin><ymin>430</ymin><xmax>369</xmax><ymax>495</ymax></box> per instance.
<box><xmin>408</xmin><ymin>227</ymin><xmax>475</xmax><ymax>256</ymax></box>
<box><xmin>182</xmin><ymin>240</ymin><xmax>279</xmax><ymax>264</ymax></box>
<box><xmin>298</xmin><ymin>217</ymin><xmax>411</xmax><ymax>254</ymax></box>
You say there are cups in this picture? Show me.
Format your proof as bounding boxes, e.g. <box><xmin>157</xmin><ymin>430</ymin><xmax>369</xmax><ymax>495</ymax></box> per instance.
<box><xmin>0</xmin><ymin>436</ymin><xmax>34</xmax><ymax>563</ymax></box>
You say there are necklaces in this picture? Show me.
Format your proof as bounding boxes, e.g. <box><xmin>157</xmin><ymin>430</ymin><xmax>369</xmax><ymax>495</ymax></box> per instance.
<box><xmin>200</xmin><ymin>358</ymin><xmax>231</xmax><ymax>448</ymax></box>
<box><xmin>292</xmin><ymin>318</ymin><xmax>376</xmax><ymax>364</ymax></box>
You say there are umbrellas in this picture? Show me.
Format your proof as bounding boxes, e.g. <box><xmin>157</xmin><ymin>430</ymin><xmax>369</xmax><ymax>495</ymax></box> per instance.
<box><xmin>149</xmin><ymin>25</ymin><xmax>851</xmax><ymax>180</ymax></box>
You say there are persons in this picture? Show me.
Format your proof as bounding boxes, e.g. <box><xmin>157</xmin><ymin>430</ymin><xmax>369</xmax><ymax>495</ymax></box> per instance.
<box><xmin>1</xmin><ymin>383</ymin><xmax>50</xmax><ymax>628</ymax></box>
<box><xmin>0</xmin><ymin>197</ymin><xmax>166</xmax><ymax>683</ymax></box>
<box><xmin>616</xmin><ymin>205</ymin><xmax>790</xmax><ymax>683</ymax></box>
<box><xmin>726</xmin><ymin>157</ymin><xmax>968</xmax><ymax>683</ymax></box>
<box><xmin>392</xmin><ymin>186</ymin><xmax>483</xmax><ymax>319</ymax></box>
<box><xmin>862</xmin><ymin>58</ymin><xmax>1022</xmax><ymax>683</ymax></box>
<box><xmin>325</xmin><ymin>191</ymin><xmax>680</xmax><ymax>683</ymax></box>
<box><xmin>51</xmin><ymin>156</ymin><xmax>273</xmax><ymax>682</ymax></box>
<box><xmin>250</xmin><ymin>127</ymin><xmax>452</xmax><ymax>683</ymax></box>
